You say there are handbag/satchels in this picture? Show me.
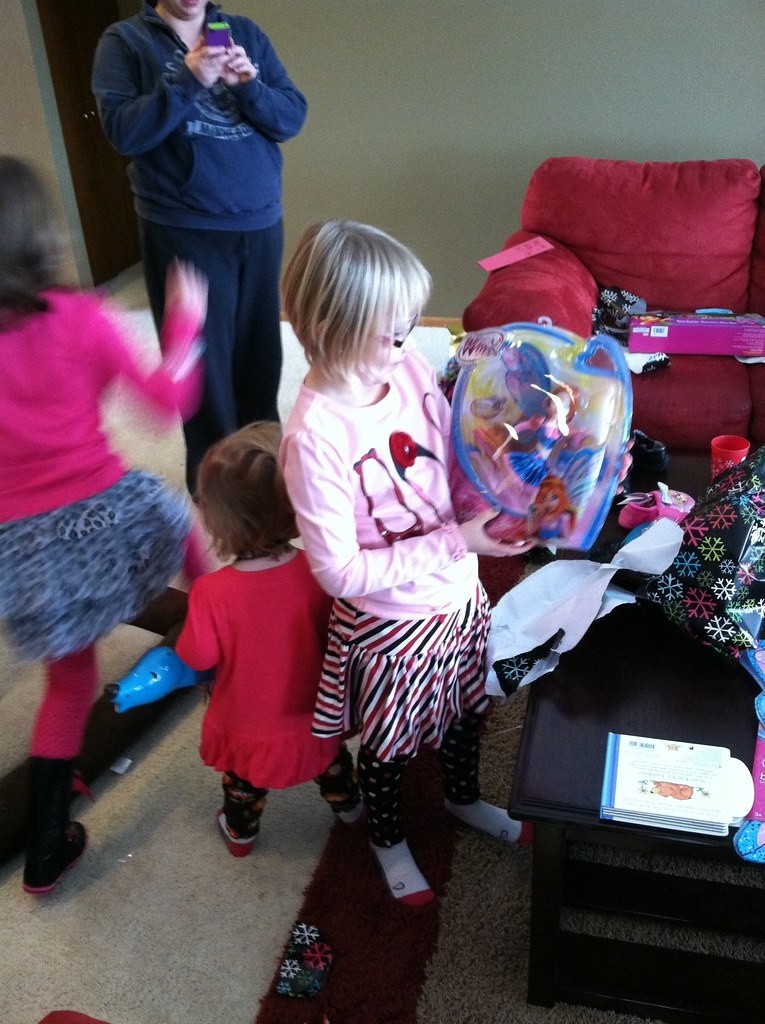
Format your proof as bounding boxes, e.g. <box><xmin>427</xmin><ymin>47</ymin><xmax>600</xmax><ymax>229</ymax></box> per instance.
<box><xmin>610</xmin><ymin>443</ymin><xmax>765</xmax><ymax>656</ymax></box>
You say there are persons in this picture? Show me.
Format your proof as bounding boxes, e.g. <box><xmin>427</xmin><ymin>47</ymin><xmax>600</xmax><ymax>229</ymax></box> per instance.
<box><xmin>491</xmin><ymin>382</ymin><xmax>584</xmax><ymax>518</ymax></box>
<box><xmin>90</xmin><ymin>1</ymin><xmax>311</xmax><ymax>499</ymax></box>
<box><xmin>175</xmin><ymin>418</ymin><xmax>366</xmax><ymax>860</ymax></box>
<box><xmin>0</xmin><ymin>149</ymin><xmax>224</xmax><ymax>899</ymax></box>
<box><xmin>279</xmin><ymin>216</ymin><xmax>536</xmax><ymax>912</ymax></box>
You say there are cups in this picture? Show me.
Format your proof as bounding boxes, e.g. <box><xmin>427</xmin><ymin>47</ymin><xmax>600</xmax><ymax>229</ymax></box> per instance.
<box><xmin>711</xmin><ymin>435</ymin><xmax>750</xmax><ymax>484</ymax></box>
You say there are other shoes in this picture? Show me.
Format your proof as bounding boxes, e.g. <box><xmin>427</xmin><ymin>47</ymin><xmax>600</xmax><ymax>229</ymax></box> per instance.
<box><xmin>336</xmin><ymin>803</ymin><xmax>367</xmax><ymax>831</ymax></box>
<box><xmin>217</xmin><ymin>811</ymin><xmax>257</xmax><ymax>857</ymax></box>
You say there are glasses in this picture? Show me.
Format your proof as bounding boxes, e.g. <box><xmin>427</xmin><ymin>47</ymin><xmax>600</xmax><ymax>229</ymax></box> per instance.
<box><xmin>370</xmin><ymin>311</ymin><xmax>420</xmax><ymax>350</ymax></box>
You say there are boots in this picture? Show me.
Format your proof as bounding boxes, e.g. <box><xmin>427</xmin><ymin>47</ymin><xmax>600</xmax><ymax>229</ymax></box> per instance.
<box><xmin>24</xmin><ymin>754</ymin><xmax>87</xmax><ymax>894</ymax></box>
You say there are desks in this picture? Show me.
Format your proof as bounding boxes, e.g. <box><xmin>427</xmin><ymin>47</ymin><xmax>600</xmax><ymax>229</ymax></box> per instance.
<box><xmin>507</xmin><ymin>603</ymin><xmax>765</xmax><ymax>1024</ymax></box>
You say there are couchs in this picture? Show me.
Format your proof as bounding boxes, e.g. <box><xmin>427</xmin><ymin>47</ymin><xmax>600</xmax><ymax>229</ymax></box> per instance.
<box><xmin>463</xmin><ymin>156</ymin><xmax>765</xmax><ymax>452</ymax></box>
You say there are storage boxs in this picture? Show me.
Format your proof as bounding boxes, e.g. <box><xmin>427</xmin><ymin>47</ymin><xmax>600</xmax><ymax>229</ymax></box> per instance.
<box><xmin>627</xmin><ymin>313</ymin><xmax>765</xmax><ymax>357</ymax></box>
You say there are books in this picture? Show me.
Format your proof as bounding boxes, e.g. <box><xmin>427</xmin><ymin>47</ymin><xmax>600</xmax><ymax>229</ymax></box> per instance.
<box><xmin>600</xmin><ymin>732</ymin><xmax>755</xmax><ymax>838</ymax></box>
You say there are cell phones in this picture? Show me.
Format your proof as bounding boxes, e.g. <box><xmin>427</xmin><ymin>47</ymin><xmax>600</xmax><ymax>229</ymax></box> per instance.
<box><xmin>207</xmin><ymin>22</ymin><xmax>230</xmax><ymax>50</ymax></box>
